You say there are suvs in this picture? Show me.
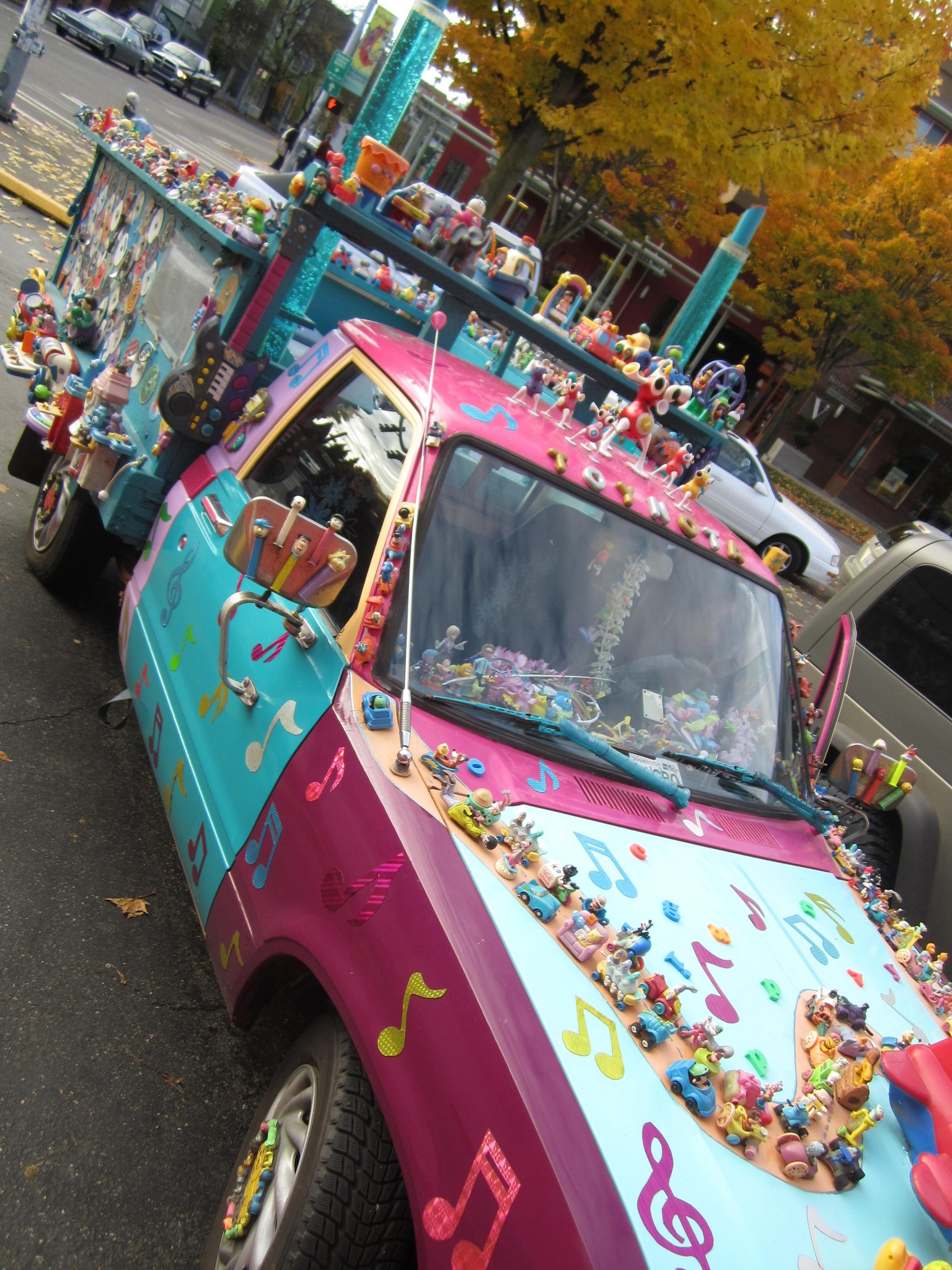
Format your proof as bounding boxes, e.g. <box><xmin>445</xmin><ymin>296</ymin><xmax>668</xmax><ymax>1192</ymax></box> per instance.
<box><xmin>117</xmin><ymin>12</ymin><xmax>170</xmax><ymax>52</ymax></box>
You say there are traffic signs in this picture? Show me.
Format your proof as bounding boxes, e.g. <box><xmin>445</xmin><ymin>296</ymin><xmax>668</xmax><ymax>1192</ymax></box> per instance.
<box><xmin>324</xmin><ymin>48</ymin><xmax>353</xmax><ymax>84</ymax></box>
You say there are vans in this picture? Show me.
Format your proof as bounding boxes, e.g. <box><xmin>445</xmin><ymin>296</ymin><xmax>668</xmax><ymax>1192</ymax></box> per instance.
<box><xmin>792</xmin><ymin>533</ymin><xmax>951</xmax><ymax>989</ymax></box>
<box><xmin>843</xmin><ymin>520</ymin><xmax>950</xmax><ymax>586</ymax></box>
<box><xmin>393</xmin><ymin>181</ymin><xmax>541</xmax><ymax>308</ymax></box>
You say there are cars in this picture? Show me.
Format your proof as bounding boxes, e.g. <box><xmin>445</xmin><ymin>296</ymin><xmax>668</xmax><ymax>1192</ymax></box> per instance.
<box><xmin>50</xmin><ymin>6</ymin><xmax>155</xmax><ymax>77</ymax></box>
<box><xmin>605</xmin><ymin>388</ymin><xmax>841</xmax><ymax>590</ymax></box>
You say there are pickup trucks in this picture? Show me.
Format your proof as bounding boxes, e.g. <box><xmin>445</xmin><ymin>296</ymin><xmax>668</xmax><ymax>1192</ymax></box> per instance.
<box><xmin>3</xmin><ymin>0</ymin><xmax>951</xmax><ymax>1269</ymax></box>
<box><xmin>145</xmin><ymin>40</ymin><xmax>223</xmax><ymax>108</ymax></box>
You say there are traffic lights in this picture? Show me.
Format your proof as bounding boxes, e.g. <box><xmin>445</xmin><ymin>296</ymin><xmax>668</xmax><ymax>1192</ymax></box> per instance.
<box><xmin>325</xmin><ymin>95</ymin><xmax>343</xmax><ymax>116</ymax></box>
